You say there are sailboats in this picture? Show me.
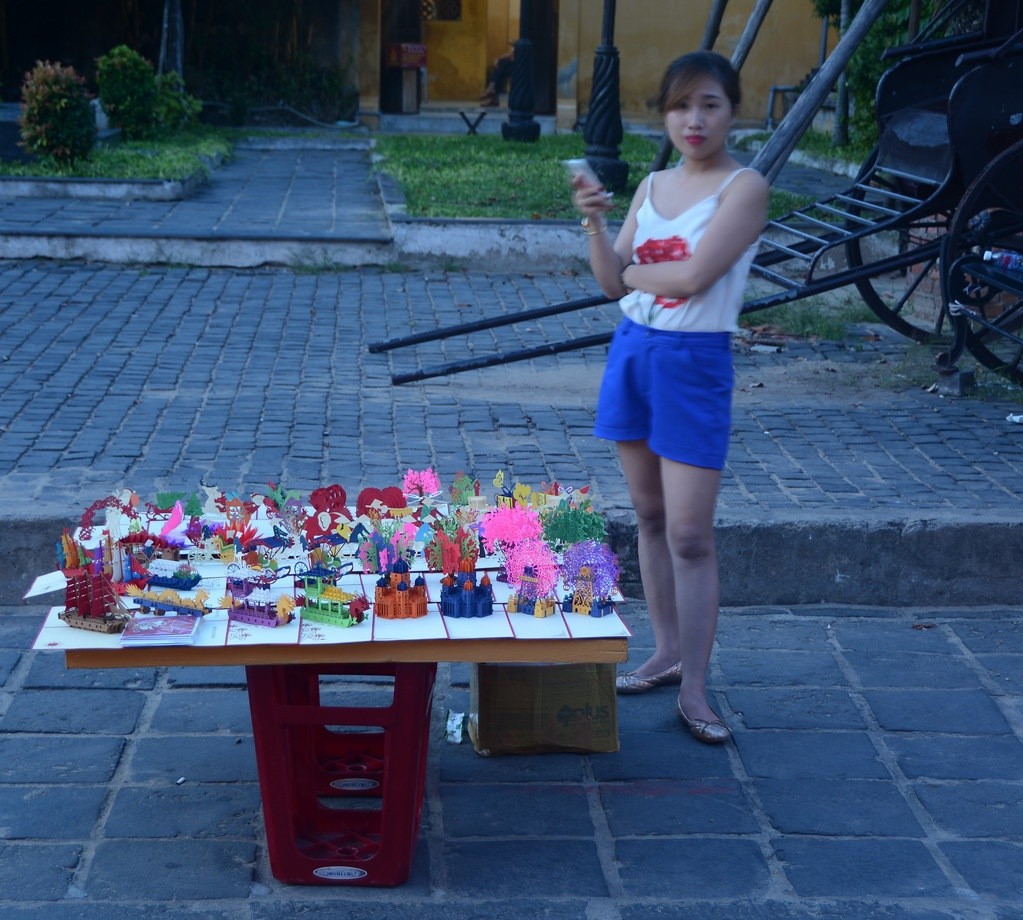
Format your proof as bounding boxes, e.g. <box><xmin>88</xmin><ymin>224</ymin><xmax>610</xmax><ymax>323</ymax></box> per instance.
<box><xmin>55</xmin><ymin>531</ymin><xmax>128</xmax><ymax>635</ymax></box>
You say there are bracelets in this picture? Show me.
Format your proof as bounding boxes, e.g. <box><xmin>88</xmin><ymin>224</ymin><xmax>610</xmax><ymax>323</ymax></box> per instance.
<box><xmin>581</xmin><ymin>217</ymin><xmax>607</xmax><ymax>236</ymax></box>
<box><xmin>619</xmin><ymin>262</ymin><xmax>636</xmax><ymax>292</ymax></box>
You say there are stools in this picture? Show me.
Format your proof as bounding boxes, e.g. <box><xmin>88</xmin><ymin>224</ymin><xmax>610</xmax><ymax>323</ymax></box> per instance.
<box><xmin>246</xmin><ymin>661</ymin><xmax>435</xmax><ymax>886</ymax></box>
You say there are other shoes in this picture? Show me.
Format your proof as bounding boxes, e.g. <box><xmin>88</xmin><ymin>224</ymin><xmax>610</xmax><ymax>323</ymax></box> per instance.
<box><xmin>675</xmin><ymin>693</ymin><xmax>732</xmax><ymax>744</ymax></box>
<box><xmin>615</xmin><ymin>661</ymin><xmax>684</xmax><ymax>695</ymax></box>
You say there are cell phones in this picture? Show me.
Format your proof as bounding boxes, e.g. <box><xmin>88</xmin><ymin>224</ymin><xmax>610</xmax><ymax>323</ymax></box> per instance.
<box><xmin>566</xmin><ymin>158</ymin><xmax>614</xmax><ymax>205</ymax></box>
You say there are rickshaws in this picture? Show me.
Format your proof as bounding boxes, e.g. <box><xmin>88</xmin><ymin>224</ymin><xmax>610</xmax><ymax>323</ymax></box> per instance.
<box><xmin>370</xmin><ymin>0</ymin><xmax>1023</xmax><ymax>390</ymax></box>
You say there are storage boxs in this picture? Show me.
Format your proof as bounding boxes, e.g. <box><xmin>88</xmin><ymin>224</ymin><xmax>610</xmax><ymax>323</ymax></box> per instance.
<box><xmin>468</xmin><ymin>659</ymin><xmax>618</xmax><ymax>757</ymax></box>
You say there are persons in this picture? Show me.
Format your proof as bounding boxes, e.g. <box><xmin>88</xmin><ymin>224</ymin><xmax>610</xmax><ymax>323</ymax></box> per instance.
<box><xmin>571</xmin><ymin>50</ymin><xmax>767</xmax><ymax>743</ymax></box>
<box><xmin>481</xmin><ymin>49</ymin><xmax>515</xmax><ymax>106</ymax></box>
